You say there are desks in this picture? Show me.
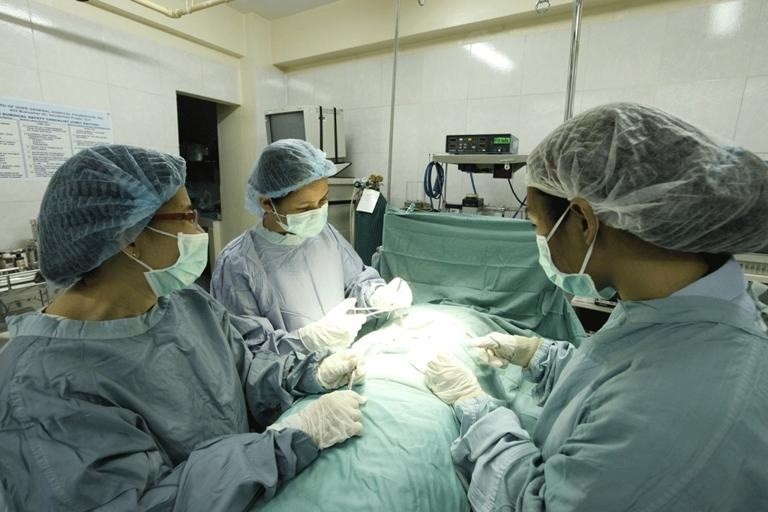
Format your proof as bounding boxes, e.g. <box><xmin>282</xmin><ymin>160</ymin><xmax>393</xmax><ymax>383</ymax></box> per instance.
<box><xmin>199</xmin><ymin>216</ymin><xmax>220</xmax><ymax>278</ymax></box>
<box><xmin>327</xmin><ymin>178</ymin><xmax>353</xmax><ymax>243</ymax></box>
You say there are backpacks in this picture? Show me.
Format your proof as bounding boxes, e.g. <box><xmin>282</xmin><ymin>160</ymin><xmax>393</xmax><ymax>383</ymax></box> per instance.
<box><xmin>297</xmin><ymin>297</ymin><xmax>368</xmax><ymax>354</ymax></box>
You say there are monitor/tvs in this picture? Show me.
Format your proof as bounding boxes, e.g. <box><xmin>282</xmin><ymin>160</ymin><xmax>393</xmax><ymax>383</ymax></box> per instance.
<box><xmin>264</xmin><ymin>104</ymin><xmax>347</xmax><ymax>159</ymax></box>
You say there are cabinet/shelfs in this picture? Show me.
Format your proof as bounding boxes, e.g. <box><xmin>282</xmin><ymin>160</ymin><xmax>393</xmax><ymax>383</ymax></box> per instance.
<box><xmin>0</xmin><ymin>268</ymin><xmax>51</xmax><ymax>327</ymax></box>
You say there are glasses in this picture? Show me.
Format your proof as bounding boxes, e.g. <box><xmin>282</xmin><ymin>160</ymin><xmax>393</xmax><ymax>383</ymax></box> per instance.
<box><xmin>148</xmin><ymin>206</ymin><xmax>200</xmax><ymax>227</ymax></box>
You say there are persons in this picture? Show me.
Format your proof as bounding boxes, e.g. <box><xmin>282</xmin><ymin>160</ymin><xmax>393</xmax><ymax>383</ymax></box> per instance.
<box><xmin>0</xmin><ymin>145</ymin><xmax>369</xmax><ymax>512</ymax></box>
<box><xmin>210</xmin><ymin>139</ymin><xmax>413</xmax><ymax>357</ymax></box>
<box><xmin>421</xmin><ymin>102</ymin><xmax>768</xmax><ymax>512</ymax></box>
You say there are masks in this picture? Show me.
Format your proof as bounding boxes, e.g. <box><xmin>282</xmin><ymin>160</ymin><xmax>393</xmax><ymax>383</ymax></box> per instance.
<box><xmin>271</xmin><ymin>199</ymin><xmax>330</xmax><ymax>241</ymax></box>
<box><xmin>534</xmin><ymin>199</ymin><xmax>620</xmax><ymax>301</ymax></box>
<box><xmin>119</xmin><ymin>225</ymin><xmax>211</xmax><ymax>299</ymax></box>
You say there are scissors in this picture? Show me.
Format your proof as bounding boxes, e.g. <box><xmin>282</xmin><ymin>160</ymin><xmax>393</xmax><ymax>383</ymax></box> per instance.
<box><xmin>353</xmin><ymin>174</ymin><xmax>383</xmax><ymax>197</ymax></box>
<box><xmin>349</xmin><ymin>306</ymin><xmax>390</xmax><ymax>318</ymax></box>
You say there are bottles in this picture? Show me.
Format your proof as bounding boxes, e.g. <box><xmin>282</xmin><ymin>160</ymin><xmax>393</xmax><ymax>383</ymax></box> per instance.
<box><xmin>0</xmin><ymin>238</ymin><xmax>41</xmax><ymax>272</ymax></box>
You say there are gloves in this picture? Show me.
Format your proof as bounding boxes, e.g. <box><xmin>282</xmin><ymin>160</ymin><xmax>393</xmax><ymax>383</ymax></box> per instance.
<box><xmin>466</xmin><ymin>330</ymin><xmax>520</xmax><ymax>371</ymax></box>
<box><xmin>265</xmin><ymin>390</ymin><xmax>366</xmax><ymax>453</ymax></box>
<box><xmin>423</xmin><ymin>352</ymin><xmax>482</xmax><ymax>410</ymax></box>
<box><xmin>315</xmin><ymin>347</ymin><xmax>367</xmax><ymax>390</ymax></box>
<box><xmin>367</xmin><ymin>275</ymin><xmax>414</xmax><ymax>319</ymax></box>
